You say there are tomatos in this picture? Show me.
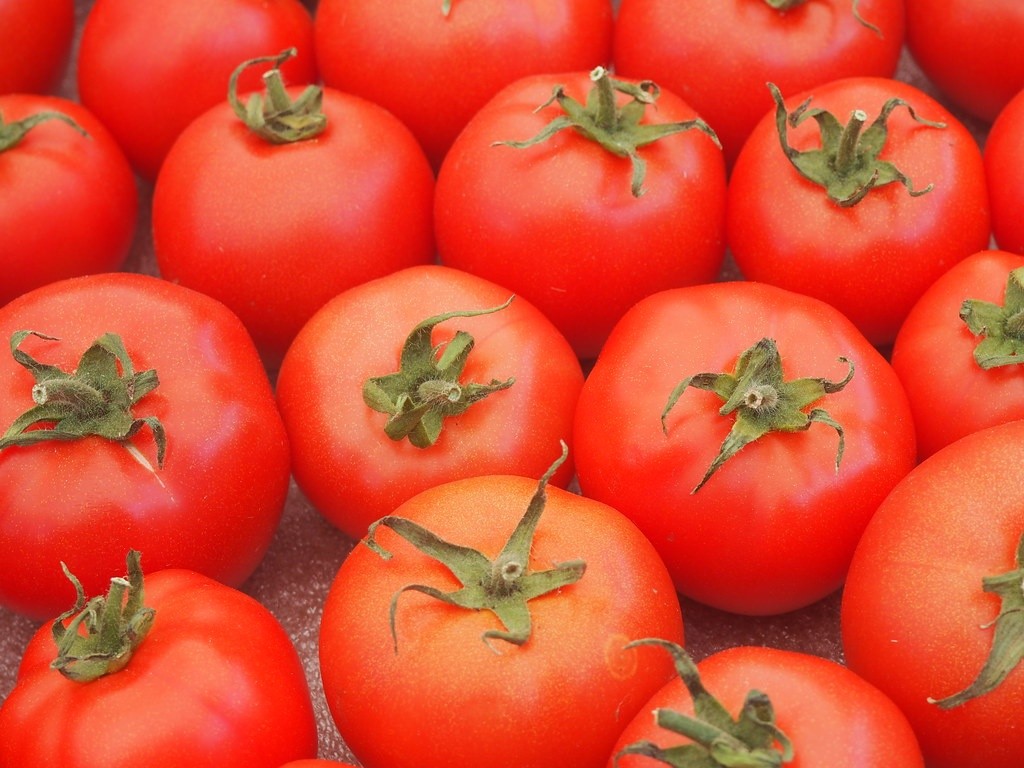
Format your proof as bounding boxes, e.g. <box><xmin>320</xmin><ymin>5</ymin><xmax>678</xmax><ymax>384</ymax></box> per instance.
<box><xmin>0</xmin><ymin>0</ymin><xmax>1024</xmax><ymax>768</ymax></box>
